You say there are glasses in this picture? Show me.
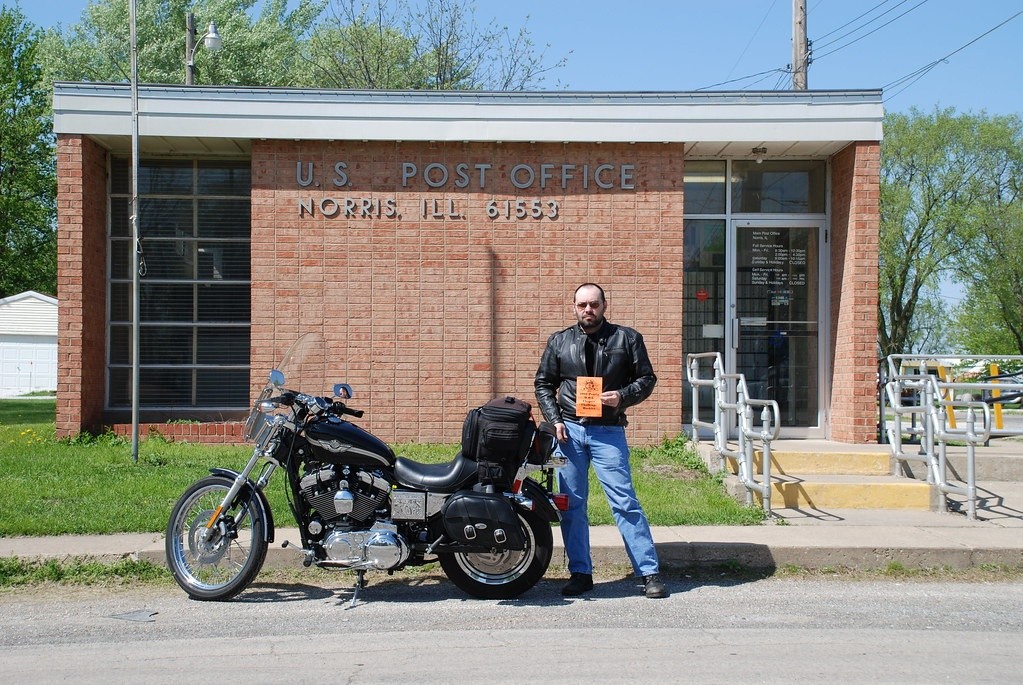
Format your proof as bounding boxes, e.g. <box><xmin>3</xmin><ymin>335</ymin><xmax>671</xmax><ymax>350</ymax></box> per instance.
<box><xmin>574</xmin><ymin>302</ymin><xmax>603</xmax><ymax>309</ymax></box>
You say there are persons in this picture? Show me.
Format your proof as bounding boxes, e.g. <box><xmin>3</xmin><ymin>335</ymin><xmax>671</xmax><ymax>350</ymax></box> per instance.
<box><xmin>534</xmin><ymin>283</ymin><xmax>668</xmax><ymax>597</ymax></box>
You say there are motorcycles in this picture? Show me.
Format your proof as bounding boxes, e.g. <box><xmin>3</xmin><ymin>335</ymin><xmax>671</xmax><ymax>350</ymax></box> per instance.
<box><xmin>165</xmin><ymin>328</ymin><xmax>570</xmax><ymax>613</ymax></box>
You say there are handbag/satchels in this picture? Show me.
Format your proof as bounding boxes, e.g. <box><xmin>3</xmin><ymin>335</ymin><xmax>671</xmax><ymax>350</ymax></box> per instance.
<box><xmin>461</xmin><ymin>396</ymin><xmax>535</xmax><ymax>461</ymax></box>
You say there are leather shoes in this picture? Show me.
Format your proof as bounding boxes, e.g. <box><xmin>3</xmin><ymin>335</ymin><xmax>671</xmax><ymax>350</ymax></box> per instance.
<box><xmin>562</xmin><ymin>572</ymin><xmax>593</xmax><ymax>596</ymax></box>
<box><xmin>642</xmin><ymin>573</ymin><xmax>666</xmax><ymax>598</ymax></box>
